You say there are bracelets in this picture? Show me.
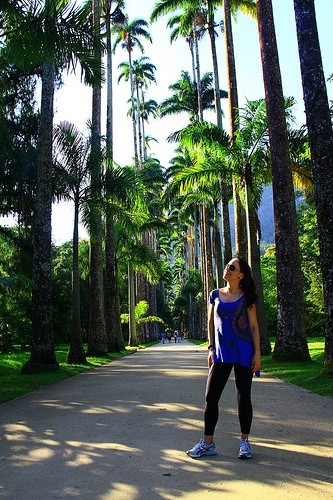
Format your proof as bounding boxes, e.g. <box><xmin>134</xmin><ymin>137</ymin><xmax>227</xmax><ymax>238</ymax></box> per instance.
<box><xmin>208</xmin><ymin>344</ymin><xmax>214</xmax><ymax>349</ymax></box>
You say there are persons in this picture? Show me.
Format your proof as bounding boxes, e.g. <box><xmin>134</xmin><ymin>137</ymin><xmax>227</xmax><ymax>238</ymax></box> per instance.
<box><xmin>186</xmin><ymin>256</ymin><xmax>261</xmax><ymax>460</ymax></box>
<box><xmin>160</xmin><ymin>330</ymin><xmax>184</xmax><ymax>344</ymax></box>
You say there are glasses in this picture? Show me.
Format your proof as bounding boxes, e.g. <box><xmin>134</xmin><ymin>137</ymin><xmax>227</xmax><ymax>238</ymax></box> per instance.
<box><xmin>226</xmin><ymin>263</ymin><xmax>241</xmax><ymax>273</ymax></box>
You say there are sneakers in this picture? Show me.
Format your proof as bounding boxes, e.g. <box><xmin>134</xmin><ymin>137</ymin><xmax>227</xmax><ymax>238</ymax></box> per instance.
<box><xmin>237</xmin><ymin>438</ymin><xmax>252</xmax><ymax>458</ymax></box>
<box><xmin>185</xmin><ymin>437</ymin><xmax>217</xmax><ymax>457</ymax></box>
